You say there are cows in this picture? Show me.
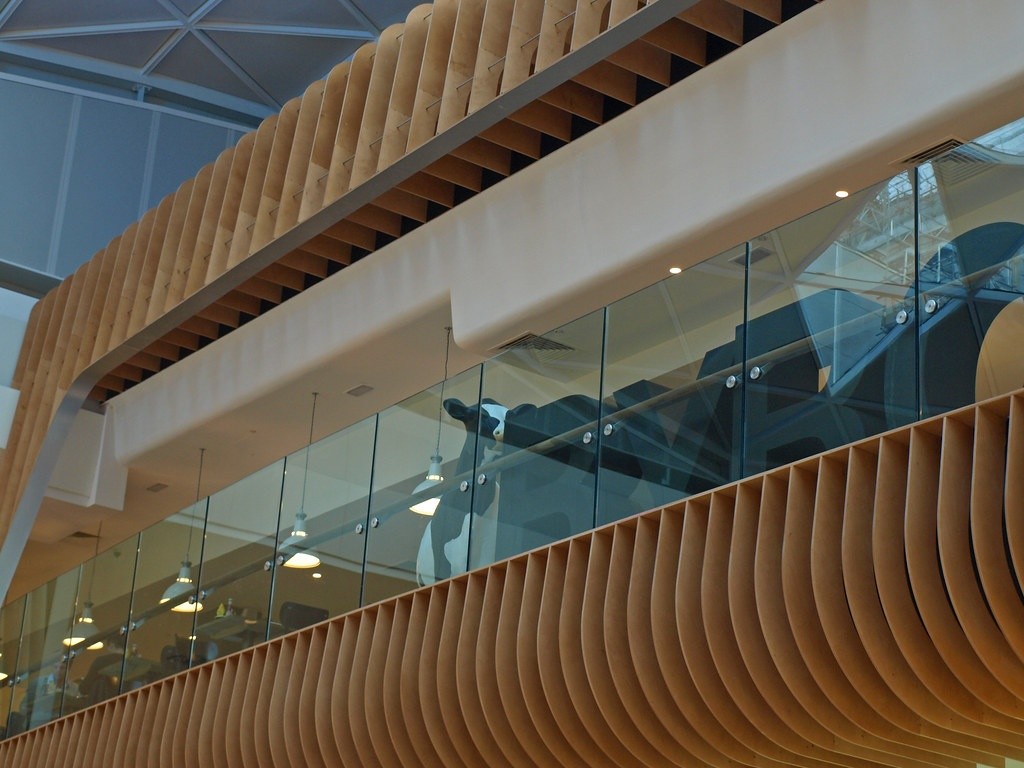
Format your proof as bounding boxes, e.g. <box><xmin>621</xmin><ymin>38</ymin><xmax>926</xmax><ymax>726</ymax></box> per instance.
<box><xmin>413</xmin><ymin>396</ymin><xmax>538</xmax><ymax>589</ymax></box>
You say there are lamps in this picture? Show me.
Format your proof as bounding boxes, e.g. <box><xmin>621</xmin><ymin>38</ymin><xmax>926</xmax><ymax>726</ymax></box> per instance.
<box><xmin>0</xmin><ymin>327</ymin><xmax>455</xmax><ymax>682</ymax></box>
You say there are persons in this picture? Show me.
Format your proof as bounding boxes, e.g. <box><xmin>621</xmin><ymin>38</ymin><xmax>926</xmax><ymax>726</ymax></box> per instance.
<box><xmin>19</xmin><ymin>656</ymin><xmax>78</xmax><ymax>731</ymax></box>
<box><xmin>79</xmin><ymin>633</ymin><xmax>141</xmax><ymax>708</ymax></box>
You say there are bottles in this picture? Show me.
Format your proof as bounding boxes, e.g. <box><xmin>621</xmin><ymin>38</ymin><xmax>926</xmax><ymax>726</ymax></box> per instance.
<box><xmin>42</xmin><ymin>677</ymin><xmax>49</xmax><ymax>694</ymax></box>
<box><xmin>226</xmin><ymin>598</ymin><xmax>233</xmax><ymax>616</ymax></box>
